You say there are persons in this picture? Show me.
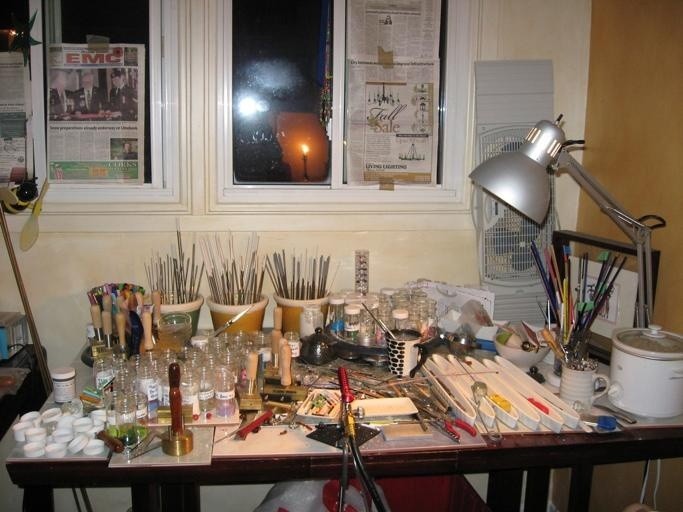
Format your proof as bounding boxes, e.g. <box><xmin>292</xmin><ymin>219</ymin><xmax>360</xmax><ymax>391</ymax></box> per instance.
<box><xmin>120</xmin><ymin>144</ymin><xmax>137</xmax><ymax>160</ymax></box>
<box><xmin>105</xmin><ymin>71</ymin><xmax>137</xmax><ymax>121</ymax></box>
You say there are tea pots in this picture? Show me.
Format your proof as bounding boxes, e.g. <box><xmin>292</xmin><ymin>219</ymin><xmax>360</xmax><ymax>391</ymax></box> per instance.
<box><xmin>299</xmin><ymin>326</ymin><xmax>339</xmax><ymax>366</ymax></box>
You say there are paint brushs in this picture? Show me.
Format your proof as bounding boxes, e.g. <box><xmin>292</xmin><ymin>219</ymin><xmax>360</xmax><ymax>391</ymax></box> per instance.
<box><xmin>211</xmin><ymin>306</ymin><xmax>254</xmax><ymax>338</ymax></box>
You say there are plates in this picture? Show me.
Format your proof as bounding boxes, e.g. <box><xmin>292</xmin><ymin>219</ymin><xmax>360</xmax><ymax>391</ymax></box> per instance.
<box><xmin>350</xmin><ymin>396</ymin><xmax>420</xmax><ymax>419</ymax></box>
<box><xmin>297</xmin><ymin>387</ymin><xmax>343</xmax><ymax>421</ymax></box>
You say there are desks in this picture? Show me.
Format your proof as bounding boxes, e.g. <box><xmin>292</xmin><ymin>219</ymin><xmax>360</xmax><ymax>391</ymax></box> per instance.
<box><xmin>9</xmin><ymin>327</ymin><xmax>682</xmax><ymax>512</ymax></box>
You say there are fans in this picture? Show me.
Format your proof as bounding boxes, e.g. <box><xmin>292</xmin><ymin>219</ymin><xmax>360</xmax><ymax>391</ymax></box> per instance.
<box><xmin>475</xmin><ymin>60</ymin><xmax>558</xmax><ymax>327</ymax></box>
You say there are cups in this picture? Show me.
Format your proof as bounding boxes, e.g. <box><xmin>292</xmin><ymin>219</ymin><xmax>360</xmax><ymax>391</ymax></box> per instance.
<box><xmin>554</xmin><ymin>329</ymin><xmax>593</xmax><ymax>377</ymax></box>
<box><xmin>380</xmin><ymin>325</ymin><xmax>426</xmax><ymax>380</ymax></box>
<box><xmin>558</xmin><ymin>358</ymin><xmax>611</xmax><ymax>415</ymax></box>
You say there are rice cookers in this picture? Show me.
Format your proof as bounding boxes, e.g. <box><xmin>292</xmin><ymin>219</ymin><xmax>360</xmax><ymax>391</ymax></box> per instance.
<box><xmin>607</xmin><ymin>324</ymin><xmax>683</xmax><ymax>419</ymax></box>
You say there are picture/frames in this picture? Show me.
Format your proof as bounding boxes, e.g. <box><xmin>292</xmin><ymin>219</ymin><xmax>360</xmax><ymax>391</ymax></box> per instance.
<box><xmin>552</xmin><ymin>231</ymin><xmax>660</xmax><ymax>366</ymax></box>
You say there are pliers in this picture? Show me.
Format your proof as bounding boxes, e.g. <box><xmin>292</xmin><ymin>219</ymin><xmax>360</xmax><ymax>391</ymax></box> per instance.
<box><xmin>437</xmin><ymin>410</ymin><xmax>477</xmax><ymax>438</ymax></box>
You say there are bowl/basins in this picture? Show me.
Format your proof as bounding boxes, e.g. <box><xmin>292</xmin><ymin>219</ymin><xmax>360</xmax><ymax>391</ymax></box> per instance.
<box><xmin>493</xmin><ymin>324</ymin><xmax>551</xmax><ymax>372</ymax></box>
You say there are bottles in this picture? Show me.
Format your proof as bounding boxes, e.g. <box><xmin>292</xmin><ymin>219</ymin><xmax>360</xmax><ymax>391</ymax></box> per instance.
<box><xmin>92</xmin><ymin>326</ymin><xmax>302</xmax><ymax>451</ymax></box>
<box><xmin>326</xmin><ymin>287</ymin><xmax>440</xmax><ymax>349</ymax></box>
<box><xmin>300</xmin><ymin>306</ymin><xmax>324</xmax><ymax>338</ymax></box>
<box><xmin>51</xmin><ymin>367</ymin><xmax>77</xmax><ymax>405</ymax></box>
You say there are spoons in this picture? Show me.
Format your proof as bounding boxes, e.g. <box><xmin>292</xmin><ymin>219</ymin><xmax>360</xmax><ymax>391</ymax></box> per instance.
<box><xmin>454</xmin><ymin>356</ymin><xmax>488</xmax><ymax>397</ymax></box>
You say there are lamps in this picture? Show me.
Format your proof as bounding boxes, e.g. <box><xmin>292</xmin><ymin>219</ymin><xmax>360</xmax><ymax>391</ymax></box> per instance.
<box><xmin>468</xmin><ymin>114</ymin><xmax>652</xmax><ymax>330</ymax></box>
